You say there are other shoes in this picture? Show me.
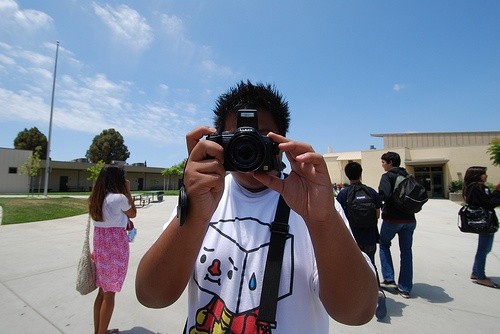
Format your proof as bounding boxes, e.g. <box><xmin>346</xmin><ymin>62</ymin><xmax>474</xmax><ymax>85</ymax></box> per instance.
<box><xmin>376</xmin><ymin>293</ymin><xmax>387</xmax><ymax>320</ymax></box>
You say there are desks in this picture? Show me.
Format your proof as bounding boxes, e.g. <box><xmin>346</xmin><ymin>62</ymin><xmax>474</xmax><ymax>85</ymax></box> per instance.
<box><xmin>132</xmin><ymin>193</ymin><xmax>141</xmax><ymax>207</ymax></box>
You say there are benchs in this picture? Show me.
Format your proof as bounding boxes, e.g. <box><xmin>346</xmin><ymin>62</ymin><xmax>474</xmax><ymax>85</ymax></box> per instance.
<box><xmin>143</xmin><ymin>197</ymin><xmax>151</xmax><ymax>206</ymax></box>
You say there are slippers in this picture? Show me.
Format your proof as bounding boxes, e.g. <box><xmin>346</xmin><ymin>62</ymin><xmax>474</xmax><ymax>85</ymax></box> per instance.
<box><xmin>471</xmin><ymin>276</ymin><xmax>491</xmax><ymax>280</ymax></box>
<box><xmin>476</xmin><ymin>282</ymin><xmax>500</xmax><ymax>288</ymax></box>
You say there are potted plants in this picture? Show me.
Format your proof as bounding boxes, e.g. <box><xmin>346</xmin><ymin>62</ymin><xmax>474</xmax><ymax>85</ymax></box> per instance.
<box><xmin>448</xmin><ymin>181</ymin><xmax>463</xmax><ymax>202</ymax></box>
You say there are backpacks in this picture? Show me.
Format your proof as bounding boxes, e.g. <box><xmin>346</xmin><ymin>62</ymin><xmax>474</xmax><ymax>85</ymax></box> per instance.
<box><xmin>389</xmin><ymin>170</ymin><xmax>428</xmax><ymax>214</ymax></box>
<box><xmin>344</xmin><ymin>184</ymin><xmax>375</xmax><ymax>226</ymax></box>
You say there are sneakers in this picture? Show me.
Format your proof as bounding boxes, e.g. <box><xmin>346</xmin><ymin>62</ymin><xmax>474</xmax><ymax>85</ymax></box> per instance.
<box><xmin>380</xmin><ymin>280</ymin><xmax>398</xmax><ymax>288</ymax></box>
<box><xmin>393</xmin><ymin>286</ymin><xmax>411</xmax><ymax>297</ymax></box>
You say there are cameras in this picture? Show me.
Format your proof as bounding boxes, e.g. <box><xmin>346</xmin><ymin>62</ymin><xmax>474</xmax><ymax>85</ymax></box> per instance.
<box><xmin>205</xmin><ymin>110</ymin><xmax>281</xmax><ymax>173</ymax></box>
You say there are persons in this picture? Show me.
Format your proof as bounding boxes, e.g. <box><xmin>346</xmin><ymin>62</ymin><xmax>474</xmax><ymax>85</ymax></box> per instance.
<box><xmin>336</xmin><ymin>162</ymin><xmax>387</xmax><ymax>320</ymax></box>
<box><xmin>87</xmin><ymin>162</ymin><xmax>136</xmax><ymax>334</ymax></box>
<box><xmin>135</xmin><ymin>78</ymin><xmax>379</xmax><ymax>334</ymax></box>
<box><xmin>462</xmin><ymin>166</ymin><xmax>500</xmax><ymax>289</ymax></box>
<box><xmin>379</xmin><ymin>152</ymin><xmax>416</xmax><ymax>297</ymax></box>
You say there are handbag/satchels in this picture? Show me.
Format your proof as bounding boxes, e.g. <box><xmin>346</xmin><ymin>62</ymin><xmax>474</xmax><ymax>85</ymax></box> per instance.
<box><xmin>76</xmin><ymin>244</ymin><xmax>98</xmax><ymax>295</ymax></box>
<box><xmin>459</xmin><ymin>204</ymin><xmax>499</xmax><ymax>233</ymax></box>
